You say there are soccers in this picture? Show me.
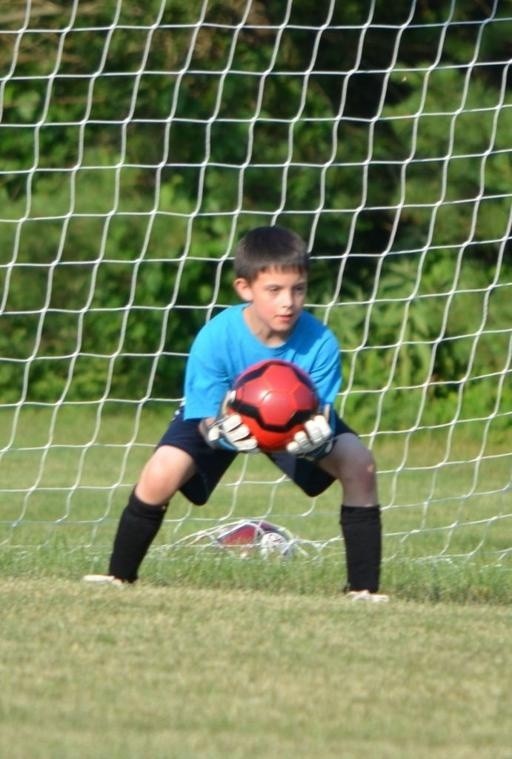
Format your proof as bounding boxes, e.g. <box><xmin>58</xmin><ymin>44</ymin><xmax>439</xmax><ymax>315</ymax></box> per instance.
<box><xmin>226</xmin><ymin>358</ymin><xmax>319</xmax><ymax>451</ymax></box>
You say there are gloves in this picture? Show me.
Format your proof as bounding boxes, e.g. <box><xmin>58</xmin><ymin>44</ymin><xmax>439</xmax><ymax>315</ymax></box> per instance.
<box><xmin>199</xmin><ymin>390</ymin><xmax>262</xmax><ymax>456</ymax></box>
<box><xmin>285</xmin><ymin>403</ymin><xmax>338</xmax><ymax>465</ymax></box>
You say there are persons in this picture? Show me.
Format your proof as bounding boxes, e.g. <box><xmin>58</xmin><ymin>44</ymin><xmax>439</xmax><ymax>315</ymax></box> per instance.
<box><xmin>85</xmin><ymin>227</ymin><xmax>388</xmax><ymax>603</ymax></box>
